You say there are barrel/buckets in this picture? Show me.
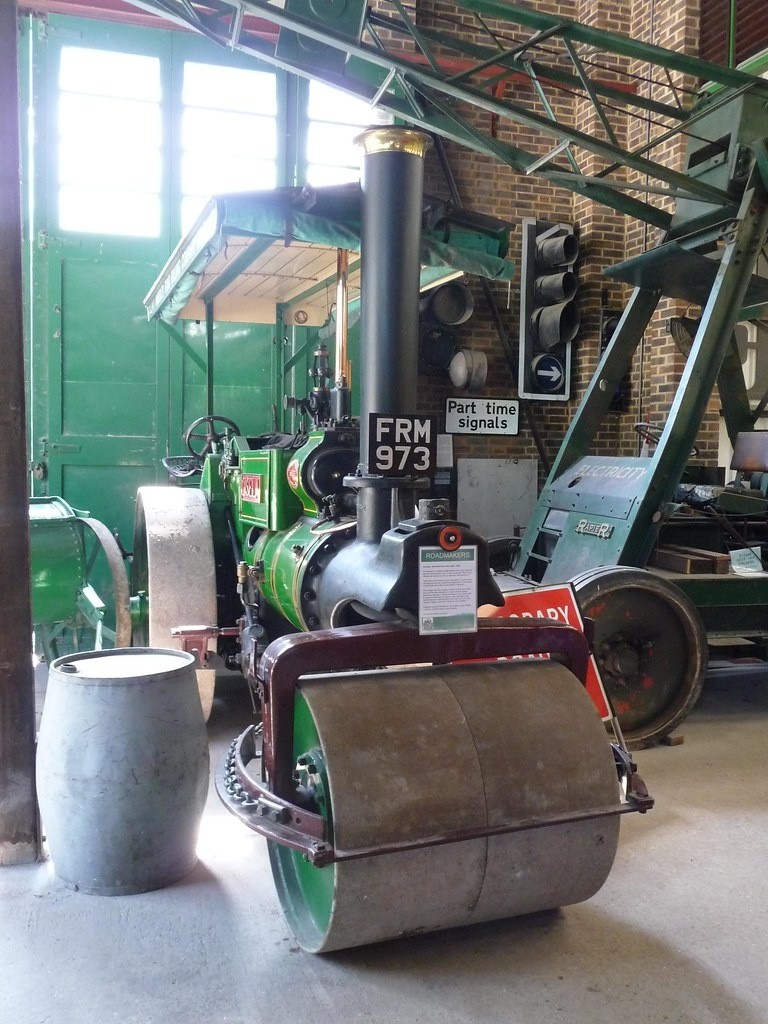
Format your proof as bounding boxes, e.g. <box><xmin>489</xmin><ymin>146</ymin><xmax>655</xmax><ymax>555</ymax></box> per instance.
<box><xmin>35</xmin><ymin>646</ymin><xmax>210</xmax><ymax>897</ymax></box>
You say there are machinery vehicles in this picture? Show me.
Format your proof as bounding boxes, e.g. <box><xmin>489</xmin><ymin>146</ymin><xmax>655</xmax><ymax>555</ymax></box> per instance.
<box><xmin>29</xmin><ymin>5</ymin><xmax>768</xmax><ymax>957</ymax></box>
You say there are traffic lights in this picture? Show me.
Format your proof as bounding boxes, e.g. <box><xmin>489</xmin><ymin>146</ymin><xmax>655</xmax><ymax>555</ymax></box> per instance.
<box><xmin>513</xmin><ymin>212</ymin><xmax>580</xmax><ymax>400</ymax></box>
